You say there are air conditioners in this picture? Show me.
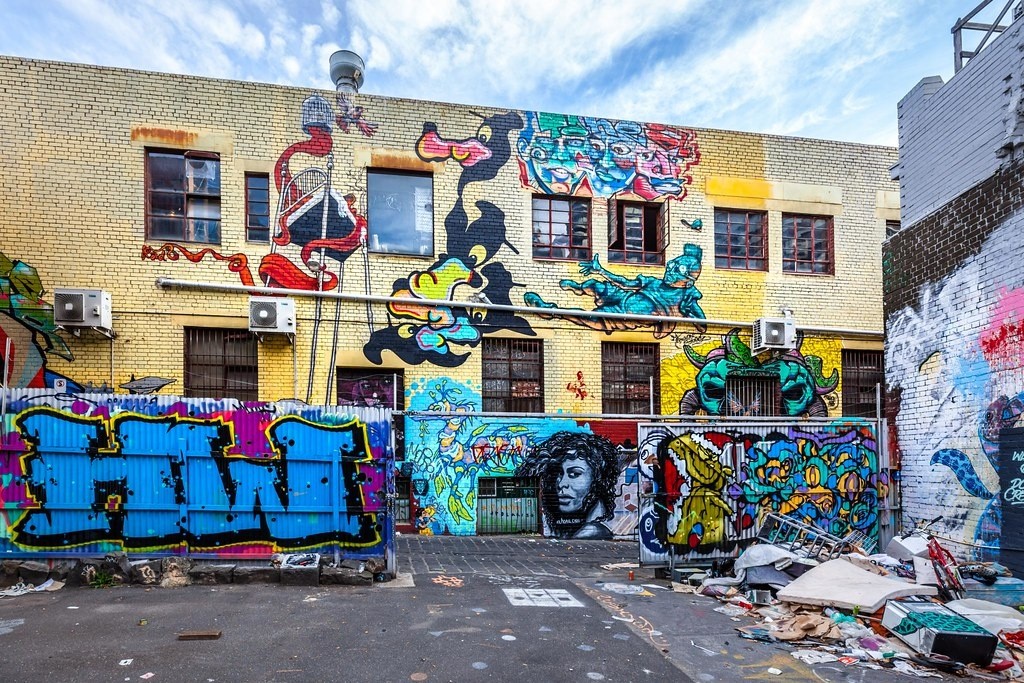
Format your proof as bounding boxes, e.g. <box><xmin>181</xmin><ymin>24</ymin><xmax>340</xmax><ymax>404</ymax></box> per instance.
<box><xmin>54</xmin><ymin>287</ymin><xmax>113</xmax><ymax>339</ymax></box>
<box><xmin>248</xmin><ymin>296</ymin><xmax>297</xmax><ymax>343</ymax></box>
<box><xmin>749</xmin><ymin>317</ymin><xmax>796</xmax><ymax>357</ymax></box>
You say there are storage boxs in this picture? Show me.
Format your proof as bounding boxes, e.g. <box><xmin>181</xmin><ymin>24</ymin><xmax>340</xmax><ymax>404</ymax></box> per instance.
<box><xmin>881</xmin><ymin>599</ymin><xmax>998</xmax><ymax>669</ymax></box>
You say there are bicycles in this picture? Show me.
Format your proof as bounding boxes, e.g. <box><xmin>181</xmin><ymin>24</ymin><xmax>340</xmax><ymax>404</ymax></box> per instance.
<box><xmin>900</xmin><ymin>516</ymin><xmax>966</xmax><ymax>602</ymax></box>
